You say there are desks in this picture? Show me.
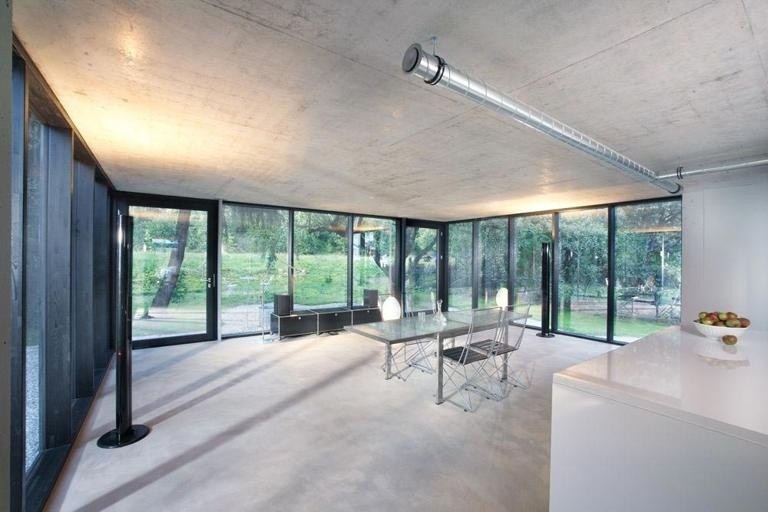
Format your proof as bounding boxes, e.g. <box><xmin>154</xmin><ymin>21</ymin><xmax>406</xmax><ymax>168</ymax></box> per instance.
<box><xmin>342</xmin><ymin>308</ymin><xmax>530</xmax><ymax>404</ymax></box>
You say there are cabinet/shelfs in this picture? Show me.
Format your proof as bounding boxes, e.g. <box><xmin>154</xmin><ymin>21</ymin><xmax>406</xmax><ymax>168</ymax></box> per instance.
<box><xmin>270</xmin><ymin>305</ymin><xmax>379</xmax><ymax>341</ymax></box>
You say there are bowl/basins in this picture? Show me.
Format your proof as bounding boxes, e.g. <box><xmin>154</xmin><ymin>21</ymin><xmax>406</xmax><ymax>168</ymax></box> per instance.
<box><xmin>697</xmin><ymin>338</ymin><xmax>749</xmax><ymax>362</ymax></box>
<box><xmin>693</xmin><ymin>318</ymin><xmax>752</xmax><ymax>339</ymax></box>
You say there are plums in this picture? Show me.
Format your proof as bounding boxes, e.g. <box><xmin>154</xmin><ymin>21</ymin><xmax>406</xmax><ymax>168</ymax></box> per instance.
<box><xmin>695</xmin><ymin>312</ymin><xmax>750</xmax><ymax>327</ymax></box>
<box><xmin>723</xmin><ymin>335</ymin><xmax>737</xmax><ymax>345</ymax></box>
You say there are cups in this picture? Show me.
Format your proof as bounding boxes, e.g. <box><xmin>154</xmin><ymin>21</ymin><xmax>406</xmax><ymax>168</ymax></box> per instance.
<box><xmin>418</xmin><ymin>312</ymin><xmax>425</xmax><ymax>321</ymax></box>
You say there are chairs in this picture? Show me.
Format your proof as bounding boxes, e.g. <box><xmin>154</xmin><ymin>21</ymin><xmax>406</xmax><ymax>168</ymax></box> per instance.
<box><xmin>377</xmin><ymin>292</ymin><xmax>532</xmax><ymax>414</ymax></box>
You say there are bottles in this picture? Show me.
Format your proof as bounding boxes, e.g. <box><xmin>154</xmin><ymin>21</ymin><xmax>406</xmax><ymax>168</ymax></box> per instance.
<box><xmin>433</xmin><ymin>299</ymin><xmax>447</xmax><ymax>321</ymax></box>
